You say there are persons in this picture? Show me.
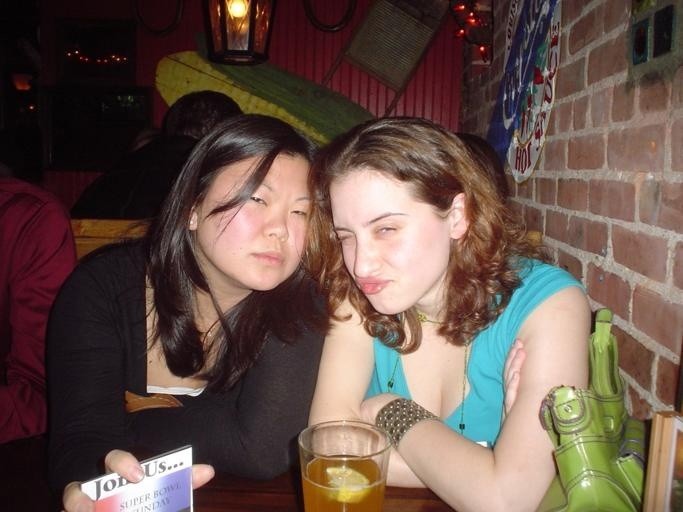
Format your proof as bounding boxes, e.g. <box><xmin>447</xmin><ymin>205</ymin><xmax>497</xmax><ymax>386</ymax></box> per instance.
<box><xmin>304</xmin><ymin>116</ymin><xmax>595</xmax><ymax>511</ymax></box>
<box><xmin>0</xmin><ymin>161</ymin><xmax>77</xmax><ymax>442</ymax></box>
<box><xmin>40</xmin><ymin>113</ymin><xmax>330</xmax><ymax>512</ymax></box>
<box><xmin>68</xmin><ymin>90</ymin><xmax>246</xmax><ymax>217</ymax></box>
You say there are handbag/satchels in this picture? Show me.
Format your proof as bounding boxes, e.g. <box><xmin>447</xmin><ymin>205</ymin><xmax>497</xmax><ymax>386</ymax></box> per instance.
<box><xmin>533</xmin><ymin>308</ymin><xmax>648</xmax><ymax>512</ymax></box>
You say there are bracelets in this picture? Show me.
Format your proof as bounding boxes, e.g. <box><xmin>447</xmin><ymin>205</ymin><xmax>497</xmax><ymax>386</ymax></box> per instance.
<box><xmin>372</xmin><ymin>398</ymin><xmax>443</xmax><ymax>449</ymax></box>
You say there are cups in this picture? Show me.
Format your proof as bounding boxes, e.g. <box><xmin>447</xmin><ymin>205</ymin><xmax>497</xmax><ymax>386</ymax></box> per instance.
<box><xmin>297</xmin><ymin>419</ymin><xmax>394</xmax><ymax>512</ymax></box>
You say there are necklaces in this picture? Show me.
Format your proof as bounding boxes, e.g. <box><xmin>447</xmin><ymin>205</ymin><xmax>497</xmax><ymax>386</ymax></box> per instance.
<box><xmin>189</xmin><ymin>285</ymin><xmax>238</xmax><ymax>340</ymax></box>
<box><xmin>387</xmin><ymin>314</ymin><xmax>468</xmax><ymax>437</ymax></box>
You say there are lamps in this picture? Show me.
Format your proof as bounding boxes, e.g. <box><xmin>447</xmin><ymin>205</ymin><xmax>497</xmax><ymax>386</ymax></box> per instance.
<box><xmin>201</xmin><ymin>1</ymin><xmax>271</xmax><ymax>68</ymax></box>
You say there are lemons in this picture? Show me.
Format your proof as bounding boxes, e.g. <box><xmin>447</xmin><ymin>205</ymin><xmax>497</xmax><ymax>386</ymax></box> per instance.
<box><xmin>324</xmin><ymin>468</ymin><xmax>370</xmax><ymax>504</ymax></box>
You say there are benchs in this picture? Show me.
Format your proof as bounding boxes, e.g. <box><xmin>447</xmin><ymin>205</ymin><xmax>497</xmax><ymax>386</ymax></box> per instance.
<box><xmin>72</xmin><ymin>220</ymin><xmax>153</xmax><ymax>270</ymax></box>
